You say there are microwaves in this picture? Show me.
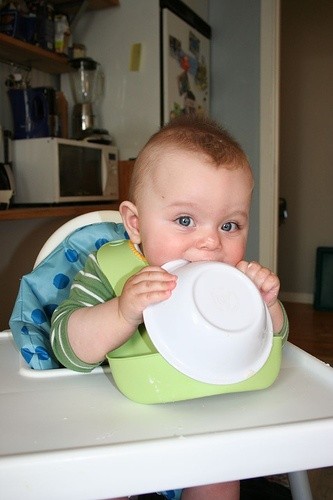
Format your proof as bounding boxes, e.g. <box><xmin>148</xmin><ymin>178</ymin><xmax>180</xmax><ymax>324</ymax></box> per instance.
<box><xmin>9</xmin><ymin>138</ymin><xmax>119</xmax><ymax>206</ymax></box>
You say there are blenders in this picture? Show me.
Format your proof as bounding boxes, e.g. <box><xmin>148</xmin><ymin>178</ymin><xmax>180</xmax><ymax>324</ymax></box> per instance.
<box><xmin>68</xmin><ymin>57</ymin><xmax>111</xmax><ymax>144</ymax></box>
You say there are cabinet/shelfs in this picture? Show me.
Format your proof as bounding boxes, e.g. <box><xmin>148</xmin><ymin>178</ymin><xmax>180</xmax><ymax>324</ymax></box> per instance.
<box><xmin>0</xmin><ymin>31</ymin><xmax>136</xmax><ymax>223</ymax></box>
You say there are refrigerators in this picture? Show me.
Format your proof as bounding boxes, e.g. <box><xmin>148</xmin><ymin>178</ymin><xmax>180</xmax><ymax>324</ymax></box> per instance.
<box><xmin>74</xmin><ymin>0</ymin><xmax>211</xmax><ymax>161</ymax></box>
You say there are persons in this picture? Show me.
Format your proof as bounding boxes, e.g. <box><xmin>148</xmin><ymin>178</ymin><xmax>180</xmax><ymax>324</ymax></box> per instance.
<box><xmin>49</xmin><ymin>110</ymin><xmax>289</xmax><ymax>500</ymax></box>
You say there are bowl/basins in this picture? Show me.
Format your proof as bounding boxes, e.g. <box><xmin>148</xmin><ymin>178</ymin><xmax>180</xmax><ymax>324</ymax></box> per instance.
<box><xmin>143</xmin><ymin>259</ymin><xmax>273</xmax><ymax>385</ymax></box>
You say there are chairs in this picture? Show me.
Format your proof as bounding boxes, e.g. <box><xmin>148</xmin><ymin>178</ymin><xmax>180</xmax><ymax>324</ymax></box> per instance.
<box><xmin>0</xmin><ymin>210</ymin><xmax>333</xmax><ymax>500</ymax></box>
